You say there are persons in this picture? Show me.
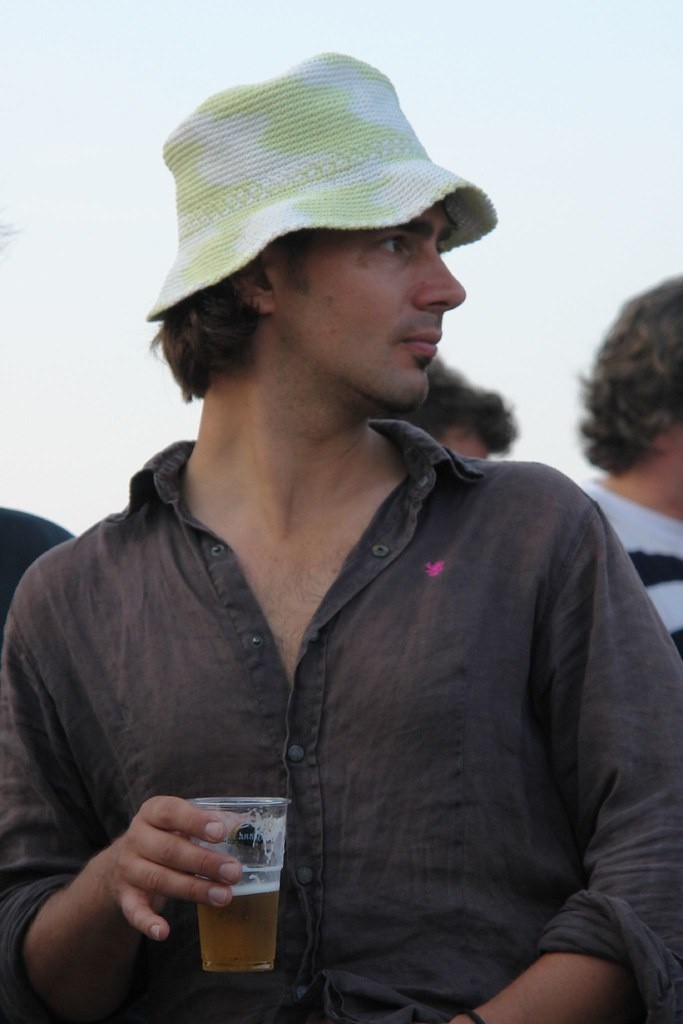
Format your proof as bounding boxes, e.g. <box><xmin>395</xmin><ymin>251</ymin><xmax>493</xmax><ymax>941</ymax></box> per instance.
<box><xmin>0</xmin><ymin>55</ymin><xmax>682</xmax><ymax>1024</ymax></box>
<box><xmin>373</xmin><ymin>272</ymin><xmax>683</xmax><ymax>652</ymax></box>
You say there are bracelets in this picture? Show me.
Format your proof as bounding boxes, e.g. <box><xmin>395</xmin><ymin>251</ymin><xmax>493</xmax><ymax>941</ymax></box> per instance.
<box><xmin>456</xmin><ymin>1007</ymin><xmax>486</xmax><ymax>1024</ymax></box>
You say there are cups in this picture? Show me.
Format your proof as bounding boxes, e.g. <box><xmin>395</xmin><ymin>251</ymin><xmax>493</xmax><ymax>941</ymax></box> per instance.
<box><xmin>186</xmin><ymin>797</ymin><xmax>291</xmax><ymax>973</ymax></box>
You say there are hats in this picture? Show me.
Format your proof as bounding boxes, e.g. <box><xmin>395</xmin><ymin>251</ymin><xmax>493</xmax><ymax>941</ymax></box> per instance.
<box><xmin>146</xmin><ymin>52</ymin><xmax>498</xmax><ymax>321</ymax></box>
